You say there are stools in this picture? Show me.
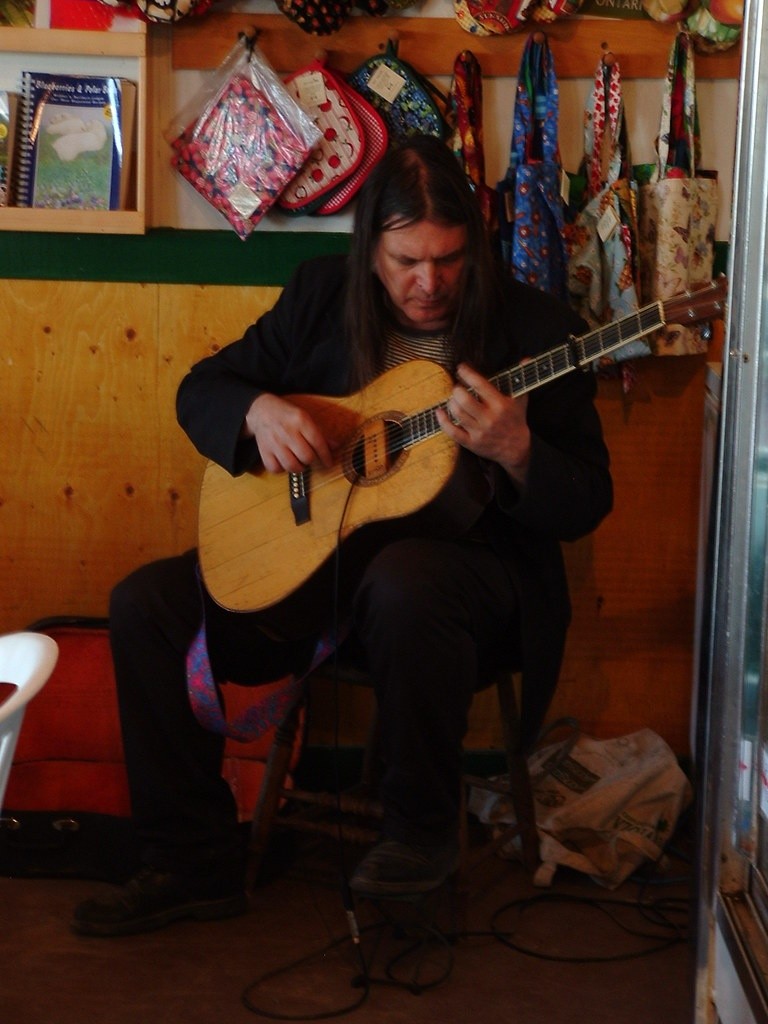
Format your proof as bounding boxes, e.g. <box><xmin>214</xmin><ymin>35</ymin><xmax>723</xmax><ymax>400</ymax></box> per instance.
<box><xmin>246</xmin><ymin>648</ymin><xmax>541</xmax><ymax>948</ymax></box>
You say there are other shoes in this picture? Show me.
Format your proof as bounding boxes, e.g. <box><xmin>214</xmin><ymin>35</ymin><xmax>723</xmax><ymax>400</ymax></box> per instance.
<box><xmin>66</xmin><ymin>840</ymin><xmax>247</xmax><ymax>934</ymax></box>
<box><xmin>351</xmin><ymin>822</ymin><xmax>458</xmax><ymax>897</ymax></box>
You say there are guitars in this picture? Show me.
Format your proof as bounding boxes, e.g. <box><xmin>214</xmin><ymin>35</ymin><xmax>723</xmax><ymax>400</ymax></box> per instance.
<box><xmin>199</xmin><ymin>266</ymin><xmax>732</xmax><ymax>617</ymax></box>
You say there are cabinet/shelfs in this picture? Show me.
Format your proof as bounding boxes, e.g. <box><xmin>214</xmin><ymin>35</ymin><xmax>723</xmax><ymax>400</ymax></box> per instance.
<box><xmin>0</xmin><ymin>0</ymin><xmax>147</xmax><ymax>235</ymax></box>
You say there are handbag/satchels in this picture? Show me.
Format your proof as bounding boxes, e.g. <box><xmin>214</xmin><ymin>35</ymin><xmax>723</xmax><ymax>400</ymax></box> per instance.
<box><xmin>466</xmin><ymin>715</ymin><xmax>690</xmax><ymax>891</ymax></box>
<box><xmin>451</xmin><ymin>32</ymin><xmax>718</xmax><ymax>371</ymax></box>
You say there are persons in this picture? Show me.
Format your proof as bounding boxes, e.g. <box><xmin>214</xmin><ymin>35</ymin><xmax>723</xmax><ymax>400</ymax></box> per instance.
<box><xmin>68</xmin><ymin>133</ymin><xmax>613</xmax><ymax>941</ymax></box>
